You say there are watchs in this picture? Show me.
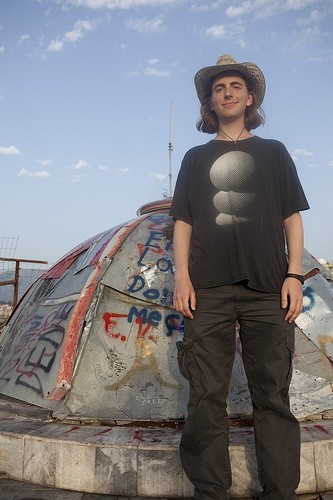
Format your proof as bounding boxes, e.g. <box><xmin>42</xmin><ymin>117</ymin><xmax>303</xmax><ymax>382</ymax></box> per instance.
<box><xmin>286</xmin><ymin>273</ymin><xmax>305</xmax><ymax>285</ymax></box>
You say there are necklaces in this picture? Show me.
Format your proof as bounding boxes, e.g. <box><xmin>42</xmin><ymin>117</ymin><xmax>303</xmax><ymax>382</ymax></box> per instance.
<box><xmin>217</xmin><ymin>123</ymin><xmax>246</xmax><ymax>144</ymax></box>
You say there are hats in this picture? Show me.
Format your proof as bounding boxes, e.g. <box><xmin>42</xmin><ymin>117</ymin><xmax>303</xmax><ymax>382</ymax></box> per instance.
<box><xmin>194</xmin><ymin>55</ymin><xmax>267</xmax><ymax>111</ymax></box>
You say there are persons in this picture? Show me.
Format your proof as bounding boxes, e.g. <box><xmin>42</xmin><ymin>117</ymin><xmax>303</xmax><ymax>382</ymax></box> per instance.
<box><xmin>168</xmin><ymin>56</ymin><xmax>310</xmax><ymax>500</ymax></box>
